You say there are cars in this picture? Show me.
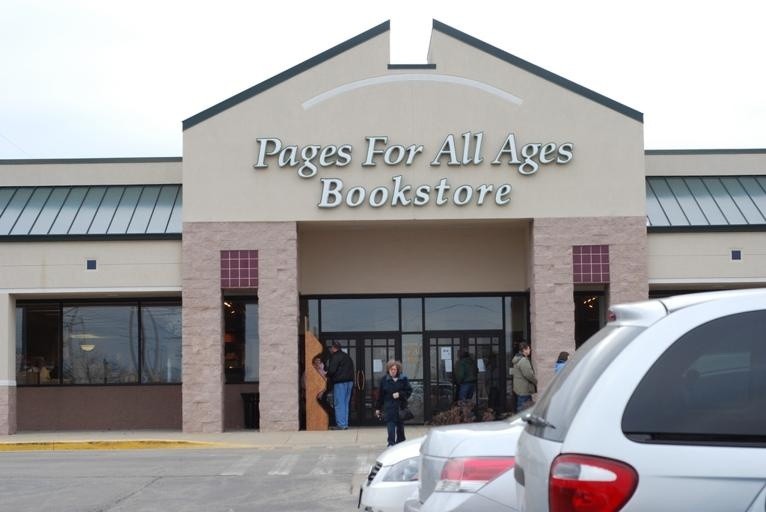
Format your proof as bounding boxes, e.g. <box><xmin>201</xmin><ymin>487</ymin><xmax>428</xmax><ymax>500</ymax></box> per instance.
<box><xmin>355</xmin><ymin>402</ymin><xmax>536</xmax><ymax>512</ymax></box>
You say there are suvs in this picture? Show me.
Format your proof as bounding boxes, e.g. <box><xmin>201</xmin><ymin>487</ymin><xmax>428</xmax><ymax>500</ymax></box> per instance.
<box><xmin>513</xmin><ymin>286</ymin><xmax>766</xmax><ymax>512</ymax></box>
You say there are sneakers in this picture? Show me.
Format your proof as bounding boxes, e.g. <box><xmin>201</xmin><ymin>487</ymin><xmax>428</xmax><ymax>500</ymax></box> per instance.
<box><xmin>330</xmin><ymin>424</ymin><xmax>347</xmax><ymax>430</ymax></box>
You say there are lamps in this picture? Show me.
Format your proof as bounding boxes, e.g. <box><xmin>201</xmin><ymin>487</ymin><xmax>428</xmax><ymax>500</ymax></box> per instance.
<box><xmin>80</xmin><ymin>344</ymin><xmax>96</xmax><ymax>353</ymax></box>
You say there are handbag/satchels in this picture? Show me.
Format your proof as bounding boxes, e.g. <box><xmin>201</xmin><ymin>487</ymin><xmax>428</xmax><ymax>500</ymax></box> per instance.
<box><xmin>393</xmin><ymin>398</ymin><xmax>414</xmax><ymax>421</ymax></box>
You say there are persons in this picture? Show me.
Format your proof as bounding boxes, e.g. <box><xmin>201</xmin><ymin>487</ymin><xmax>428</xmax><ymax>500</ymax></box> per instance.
<box><xmin>453</xmin><ymin>351</ymin><xmax>478</xmax><ymax>400</ymax></box>
<box><xmin>314</xmin><ymin>356</ymin><xmax>324</xmax><ymax>372</ymax></box>
<box><xmin>327</xmin><ymin>341</ymin><xmax>353</xmax><ymax>429</ymax></box>
<box><xmin>511</xmin><ymin>342</ymin><xmax>538</xmax><ymax>411</ymax></box>
<box><xmin>374</xmin><ymin>359</ymin><xmax>413</xmax><ymax>445</ymax></box>
<box><xmin>554</xmin><ymin>352</ymin><xmax>569</xmax><ymax>373</ymax></box>
